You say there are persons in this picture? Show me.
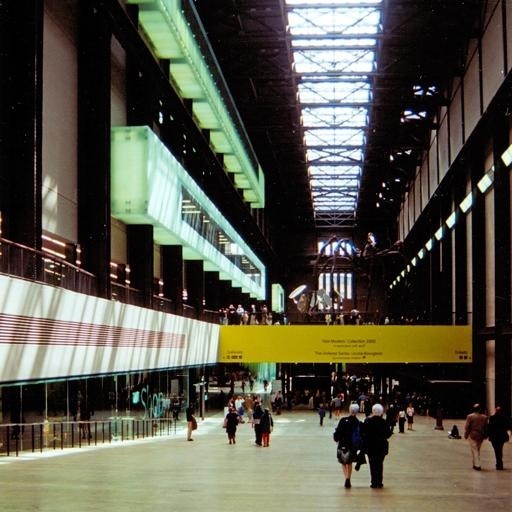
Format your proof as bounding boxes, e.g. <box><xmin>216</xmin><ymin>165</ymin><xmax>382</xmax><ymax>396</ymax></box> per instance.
<box><xmin>333</xmin><ymin>403</ymin><xmax>363</xmax><ymax>488</ymax></box>
<box><xmin>464</xmin><ymin>403</ymin><xmax>489</xmax><ymax>470</ymax></box>
<box><xmin>172</xmin><ymin>372</ymin><xmax>415</xmax><ymax>447</ymax></box>
<box><xmin>363</xmin><ymin>403</ymin><xmax>392</xmax><ymax>488</ymax></box>
<box><xmin>218</xmin><ymin>304</ymin><xmax>289</xmax><ymax>325</ymax></box>
<box><xmin>488</xmin><ymin>406</ymin><xmax>510</xmax><ymax>470</ymax></box>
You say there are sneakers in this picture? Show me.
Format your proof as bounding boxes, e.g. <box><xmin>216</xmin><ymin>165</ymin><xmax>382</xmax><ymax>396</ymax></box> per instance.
<box><xmin>344</xmin><ymin>478</ymin><xmax>352</xmax><ymax>488</ymax></box>
<box><xmin>369</xmin><ymin>483</ymin><xmax>384</xmax><ymax>489</ymax></box>
<box><xmin>472</xmin><ymin>464</ymin><xmax>504</xmax><ymax>471</ymax></box>
